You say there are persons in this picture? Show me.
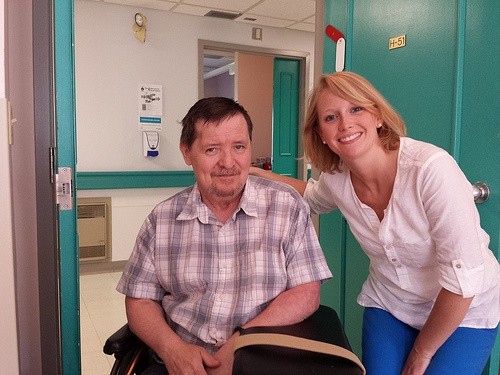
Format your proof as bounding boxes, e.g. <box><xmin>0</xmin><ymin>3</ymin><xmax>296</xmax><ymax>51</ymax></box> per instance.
<box><xmin>115</xmin><ymin>96</ymin><xmax>334</xmax><ymax>375</ymax></box>
<box><xmin>250</xmin><ymin>72</ymin><xmax>500</xmax><ymax>375</ymax></box>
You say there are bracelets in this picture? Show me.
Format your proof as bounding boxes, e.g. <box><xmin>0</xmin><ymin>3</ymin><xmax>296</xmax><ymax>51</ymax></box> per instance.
<box><xmin>235</xmin><ymin>326</ymin><xmax>244</xmax><ymax>334</ymax></box>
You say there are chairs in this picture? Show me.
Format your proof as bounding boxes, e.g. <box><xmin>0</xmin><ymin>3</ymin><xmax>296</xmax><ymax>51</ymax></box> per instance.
<box><xmin>102</xmin><ymin>304</ymin><xmax>367</xmax><ymax>375</ymax></box>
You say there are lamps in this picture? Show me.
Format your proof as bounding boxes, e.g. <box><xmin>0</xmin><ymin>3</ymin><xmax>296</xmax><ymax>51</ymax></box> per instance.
<box><xmin>133</xmin><ymin>12</ymin><xmax>148</xmax><ymax>43</ymax></box>
